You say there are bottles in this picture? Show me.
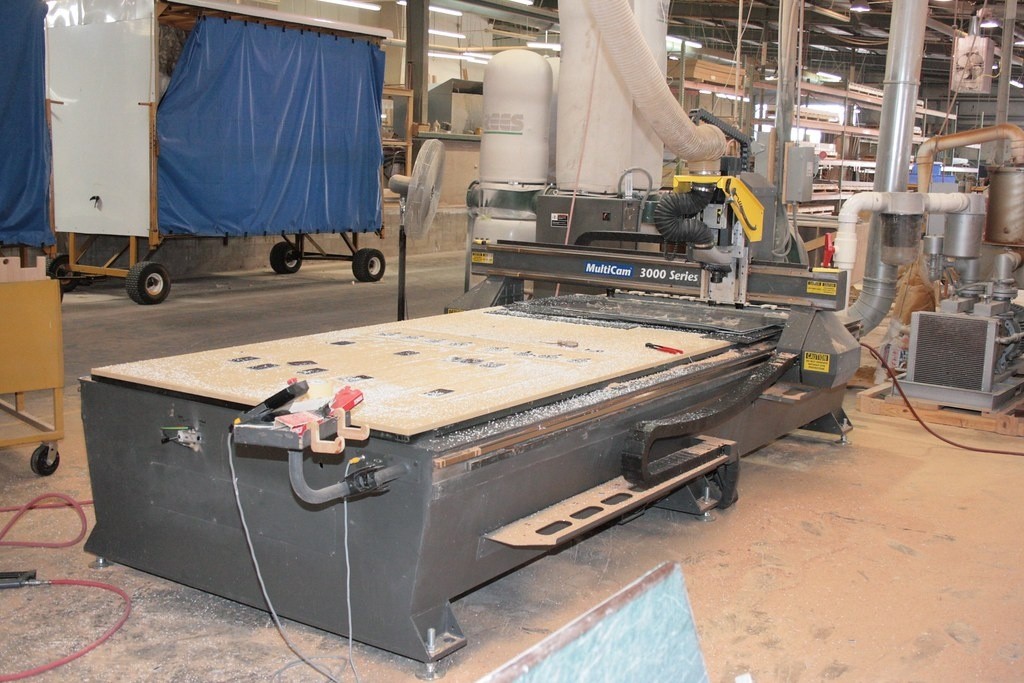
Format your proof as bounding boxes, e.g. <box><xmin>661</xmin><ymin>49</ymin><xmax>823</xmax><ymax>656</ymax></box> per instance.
<box><xmin>432</xmin><ymin>120</ymin><xmax>441</xmax><ymax>132</ymax></box>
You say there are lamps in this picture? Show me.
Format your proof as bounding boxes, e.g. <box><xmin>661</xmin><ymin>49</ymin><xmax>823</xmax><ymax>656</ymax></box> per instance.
<box><xmin>318</xmin><ymin>0</ymin><xmax>382</xmax><ymax>11</ymax></box>
<box><xmin>396</xmin><ymin>0</ymin><xmax>494</xmax><ymax>64</ymax></box>
<box><xmin>849</xmin><ymin>0</ymin><xmax>872</xmax><ymax>12</ymax></box>
<box><xmin>666</xmin><ymin>0</ymin><xmax>703</xmax><ymax>49</ymax></box>
<box><xmin>526</xmin><ymin>30</ymin><xmax>561</xmax><ymax>51</ymax></box>
<box><xmin>816</xmin><ymin>46</ymin><xmax>842</xmax><ymax>80</ymax></box>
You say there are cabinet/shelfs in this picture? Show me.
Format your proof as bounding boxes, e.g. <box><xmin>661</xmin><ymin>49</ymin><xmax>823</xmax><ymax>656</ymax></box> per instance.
<box><xmin>661</xmin><ymin>39</ymin><xmax>985</xmax><ymax>214</ymax></box>
<box><xmin>381</xmin><ymin>87</ymin><xmax>414</xmax><ymax>199</ymax></box>
